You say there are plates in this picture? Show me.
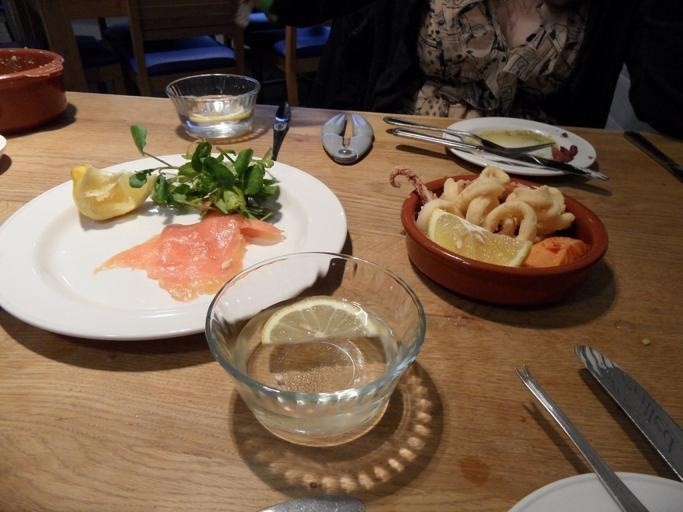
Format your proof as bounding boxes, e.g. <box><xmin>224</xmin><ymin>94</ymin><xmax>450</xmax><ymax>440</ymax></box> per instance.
<box><xmin>500</xmin><ymin>469</ymin><xmax>683</xmax><ymax>511</ymax></box>
<box><xmin>445</xmin><ymin>115</ymin><xmax>598</xmax><ymax>178</ymax></box>
<box><xmin>1</xmin><ymin>151</ymin><xmax>349</xmax><ymax>344</ymax></box>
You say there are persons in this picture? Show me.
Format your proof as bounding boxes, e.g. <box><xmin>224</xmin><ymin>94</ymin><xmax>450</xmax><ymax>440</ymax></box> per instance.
<box><xmin>270</xmin><ymin>0</ymin><xmax>427</xmax><ymax>114</ymax></box>
<box><xmin>414</xmin><ymin>0</ymin><xmax>590</xmax><ymax>126</ymax></box>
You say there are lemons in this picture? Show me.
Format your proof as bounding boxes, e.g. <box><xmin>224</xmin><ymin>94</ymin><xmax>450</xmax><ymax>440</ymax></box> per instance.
<box><xmin>260</xmin><ymin>295</ymin><xmax>379</xmax><ymax>345</ymax></box>
<box><xmin>427</xmin><ymin>208</ymin><xmax>533</xmax><ymax>267</ymax></box>
<box><xmin>70</xmin><ymin>165</ymin><xmax>158</xmax><ymax>221</ymax></box>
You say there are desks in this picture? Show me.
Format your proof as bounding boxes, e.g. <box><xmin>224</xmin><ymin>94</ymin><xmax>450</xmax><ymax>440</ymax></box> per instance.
<box><xmin>1</xmin><ymin>1</ymin><xmax>128</xmax><ymax>96</ymax></box>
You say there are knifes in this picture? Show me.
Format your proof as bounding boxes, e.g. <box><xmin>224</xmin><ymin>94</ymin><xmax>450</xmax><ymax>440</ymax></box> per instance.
<box><xmin>391</xmin><ymin>127</ymin><xmax>613</xmax><ymax>183</ymax></box>
<box><xmin>575</xmin><ymin>345</ymin><xmax>682</xmax><ymax>482</ymax></box>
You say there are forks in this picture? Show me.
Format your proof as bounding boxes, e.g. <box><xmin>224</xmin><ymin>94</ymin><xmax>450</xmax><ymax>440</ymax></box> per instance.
<box><xmin>382</xmin><ymin>116</ymin><xmax>556</xmax><ymax>153</ymax></box>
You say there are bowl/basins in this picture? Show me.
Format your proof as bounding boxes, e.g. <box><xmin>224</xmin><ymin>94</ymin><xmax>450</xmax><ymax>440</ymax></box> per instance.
<box><xmin>0</xmin><ymin>44</ymin><xmax>76</xmax><ymax>135</ymax></box>
<box><xmin>399</xmin><ymin>175</ymin><xmax>608</xmax><ymax>306</ymax></box>
<box><xmin>164</xmin><ymin>73</ymin><xmax>260</xmax><ymax>141</ymax></box>
<box><xmin>204</xmin><ymin>252</ymin><xmax>428</xmax><ymax>449</ymax></box>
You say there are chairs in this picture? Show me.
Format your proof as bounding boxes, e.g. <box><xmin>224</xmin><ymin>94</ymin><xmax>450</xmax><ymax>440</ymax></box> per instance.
<box><xmin>223</xmin><ymin>10</ymin><xmax>338</xmax><ymax>109</ymax></box>
<box><xmin>104</xmin><ymin>0</ymin><xmax>250</xmax><ymax>100</ymax></box>
